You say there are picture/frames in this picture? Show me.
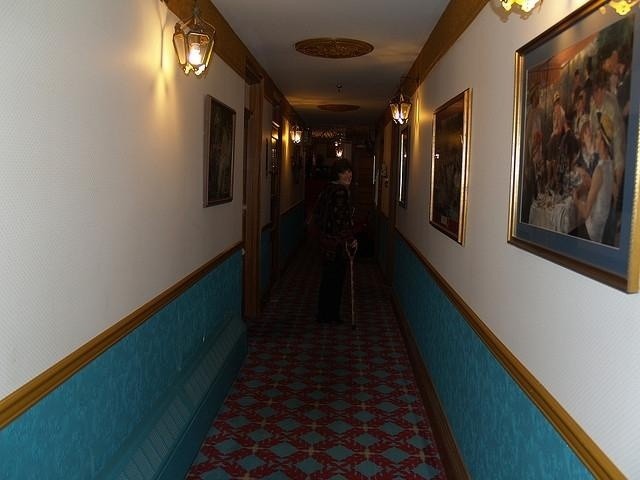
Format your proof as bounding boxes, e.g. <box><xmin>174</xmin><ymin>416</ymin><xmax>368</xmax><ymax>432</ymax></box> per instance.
<box><xmin>507</xmin><ymin>0</ymin><xmax>640</xmax><ymax>294</ymax></box>
<box><xmin>399</xmin><ymin>125</ymin><xmax>410</xmax><ymax>209</ymax></box>
<box><xmin>428</xmin><ymin>87</ymin><xmax>473</xmax><ymax>246</ymax></box>
<box><xmin>202</xmin><ymin>94</ymin><xmax>237</xmax><ymax>209</ymax></box>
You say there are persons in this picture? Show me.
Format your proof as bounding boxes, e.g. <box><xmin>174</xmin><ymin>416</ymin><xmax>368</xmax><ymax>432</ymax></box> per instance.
<box><xmin>522</xmin><ymin>39</ymin><xmax>633</xmax><ymax>248</ymax></box>
<box><xmin>312</xmin><ymin>159</ymin><xmax>365</xmax><ymax>325</ymax></box>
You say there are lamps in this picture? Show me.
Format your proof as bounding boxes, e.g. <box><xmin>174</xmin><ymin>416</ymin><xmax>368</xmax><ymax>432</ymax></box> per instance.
<box><xmin>172</xmin><ymin>0</ymin><xmax>216</xmax><ymax>80</ymax></box>
<box><xmin>388</xmin><ymin>72</ymin><xmax>419</xmax><ymax>126</ymax></box>
<box><xmin>290</xmin><ymin>119</ymin><xmax>303</xmax><ymax>144</ymax></box>
